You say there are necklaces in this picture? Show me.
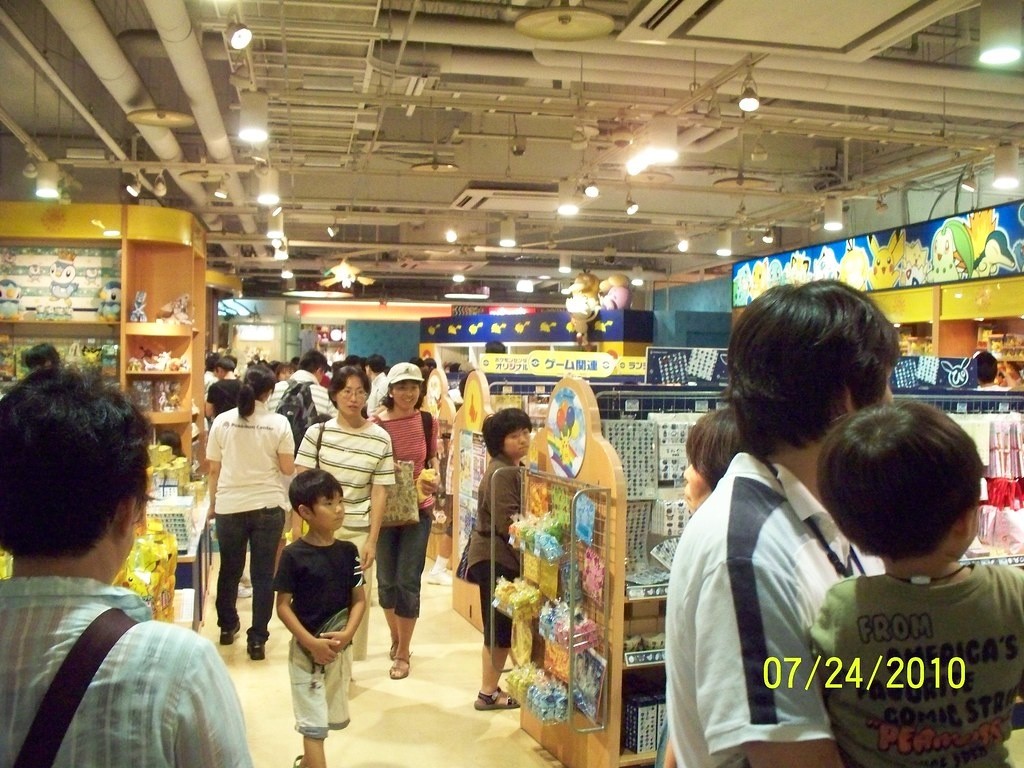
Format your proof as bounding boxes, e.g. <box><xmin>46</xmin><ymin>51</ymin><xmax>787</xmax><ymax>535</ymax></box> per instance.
<box><xmin>885</xmin><ymin>565</ymin><xmax>968</xmax><ymax>584</ymax></box>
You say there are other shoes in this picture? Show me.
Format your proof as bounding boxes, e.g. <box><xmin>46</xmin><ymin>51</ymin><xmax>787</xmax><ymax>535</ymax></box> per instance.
<box><xmin>246</xmin><ymin>639</ymin><xmax>265</xmax><ymax>659</ymax></box>
<box><xmin>219</xmin><ymin>621</ymin><xmax>240</xmax><ymax>644</ymax></box>
<box><xmin>238</xmin><ymin>586</ymin><xmax>253</xmax><ymax>597</ymax></box>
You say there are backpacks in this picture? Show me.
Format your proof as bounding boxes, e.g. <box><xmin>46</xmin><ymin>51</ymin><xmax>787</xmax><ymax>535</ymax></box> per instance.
<box><xmin>275</xmin><ymin>377</ymin><xmax>319</xmax><ymax>457</ymax></box>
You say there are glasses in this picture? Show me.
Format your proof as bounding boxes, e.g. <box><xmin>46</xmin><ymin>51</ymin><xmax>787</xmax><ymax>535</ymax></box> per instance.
<box><xmin>340</xmin><ymin>390</ymin><xmax>367</xmax><ymax>399</ymax></box>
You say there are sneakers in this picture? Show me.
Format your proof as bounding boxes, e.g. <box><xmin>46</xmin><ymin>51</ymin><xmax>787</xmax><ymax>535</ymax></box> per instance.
<box><xmin>428</xmin><ymin>568</ymin><xmax>452</xmax><ymax>585</ymax></box>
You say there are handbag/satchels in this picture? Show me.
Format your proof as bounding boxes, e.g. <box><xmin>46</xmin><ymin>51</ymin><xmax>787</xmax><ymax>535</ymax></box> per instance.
<box><xmin>454</xmin><ymin>516</ymin><xmax>481</xmax><ymax>585</ymax></box>
<box><xmin>369</xmin><ymin>415</ymin><xmax>420</xmax><ymax>527</ymax></box>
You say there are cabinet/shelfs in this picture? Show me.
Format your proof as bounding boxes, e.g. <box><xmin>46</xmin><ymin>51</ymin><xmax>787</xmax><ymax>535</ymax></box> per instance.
<box><xmin>452</xmin><ymin>368</ymin><xmax>674</xmax><ymax>768</ymax></box>
<box><xmin>0</xmin><ymin>201</ymin><xmax>208</xmax><ymax>472</ymax></box>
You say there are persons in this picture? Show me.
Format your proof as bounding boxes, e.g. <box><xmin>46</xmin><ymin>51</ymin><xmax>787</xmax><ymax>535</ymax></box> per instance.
<box><xmin>268</xmin><ymin>469</ymin><xmax>368</xmax><ymax>768</ymax></box>
<box><xmin>665</xmin><ymin>278</ymin><xmax>899</xmax><ymax>768</ymax></box>
<box><xmin>24</xmin><ymin>343</ymin><xmax>60</xmax><ymax>371</ymax></box>
<box><xmin>201</xmin><ymin>350</ymin><xmax>462</xmax><ymax>681</ymax></box>
<box><xmin>467</xmin><ymin>408</ymin><xmax>532</xmax><ymax>711</ymax></box>
<box><xmin>0</xmin><ymin>363</ymin><xmax>252</xmax><ymax>768</ymax></box>
<box><xmin>811</xmin><ymin>402</ymin><xmax>1024</xmax><ymax>768</ymax></box>
<box><xmin>973</xmin><ymin>350</ymin><xmax>1016</xmax><ymax>393</ymax></box>
<box><xmin>460</xmin><ymin>341</ymin><xmax>506</xmax><ymax>400</ymax></box>
<box><xmin>157</xmin><ymin>430</ymin><xmax>193</xmax><ymax>481</ymax></box>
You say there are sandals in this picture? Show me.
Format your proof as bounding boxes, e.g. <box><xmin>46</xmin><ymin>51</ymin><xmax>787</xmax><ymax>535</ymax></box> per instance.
<box><xmin>390</xmin><ymin>643</ymin><xmax>397</xmax><ymax>658</ymax></box>
<box><xmin>475</xmin><ymin>687</ymin><xmax>520</xmax><ymax>710</ymax></box>
<box><xmin>390</xmin><ymin>655</ymin><xmax>411</xmax><ymax>679</ymax></box>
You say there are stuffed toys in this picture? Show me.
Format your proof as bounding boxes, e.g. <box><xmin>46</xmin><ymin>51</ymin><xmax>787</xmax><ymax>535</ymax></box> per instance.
<box><xmin>561</xmin><ymin>269</ymin><xmax>632</xmax><ymax>354</ymax></box>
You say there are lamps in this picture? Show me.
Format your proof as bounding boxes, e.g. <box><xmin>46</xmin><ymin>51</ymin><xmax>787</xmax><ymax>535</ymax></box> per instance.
<box><xmin>991</xmin><ymin>131</ymin><xmax>1020</xmax><ymax>190</ymax></box>
<box><xmin>962</xmin><ymin>160</ymin><xmax>977</xmax><ymax>192</ymax></box>
<box><xmin>22</xmin><ymin>18</ymin><xmax>842</xmax><ymax>289</ymax></box>
<box><xmin>875</xmin><ymin>194</ymin><xmax>888</xmax><ymax>213</ymax></box>
<box><xmin>977</xmin><ymin>0</ymin><xmax>1024</xmax><ymax>67</ymax></box>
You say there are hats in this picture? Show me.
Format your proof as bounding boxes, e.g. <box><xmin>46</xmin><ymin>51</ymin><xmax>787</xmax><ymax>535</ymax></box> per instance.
<box><xmin>387</xmin><ymin>362</ymin><xmax>425</xmax><ymax>385</ymax></box>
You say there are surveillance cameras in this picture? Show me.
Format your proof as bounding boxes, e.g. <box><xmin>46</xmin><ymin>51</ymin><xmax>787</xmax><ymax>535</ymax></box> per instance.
<box><xmin>603</xmin><ymin>246</ymin><xmax>616</xmax><ymax>263</ymax></box>
<box><xmin>508</xmin><ymin>138</ymin><xmax>527</xmax><ymax>155</ymax></box>
